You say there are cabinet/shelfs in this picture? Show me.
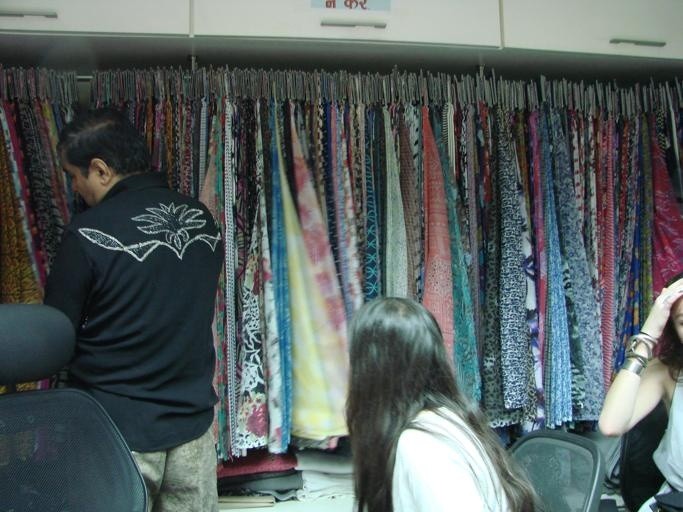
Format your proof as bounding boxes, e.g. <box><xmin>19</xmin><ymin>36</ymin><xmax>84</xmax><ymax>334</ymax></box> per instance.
<box><xmin>0</xmin><ymin>1</ymin><xmax>682</xmax><ymax>74</ymax></box>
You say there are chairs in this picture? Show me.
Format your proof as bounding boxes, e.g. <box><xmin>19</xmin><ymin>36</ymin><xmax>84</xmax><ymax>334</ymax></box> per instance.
<box><xmin>508</xmin><ymin>431</ymin><xmax>605</xmax><ymax>512</ymax></box>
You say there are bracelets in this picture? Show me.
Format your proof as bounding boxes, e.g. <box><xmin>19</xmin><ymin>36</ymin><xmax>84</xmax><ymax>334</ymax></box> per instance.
<box><xmin>620</xmin><ymin>331</ymin><xmax>660</xmax><ymax>378</ymax></box>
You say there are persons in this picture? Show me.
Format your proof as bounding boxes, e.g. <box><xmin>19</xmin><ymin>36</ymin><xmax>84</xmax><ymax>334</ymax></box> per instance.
<box><xmin>43</xmin><ymin>105</ymin><xmax>223</xmax><ymax>511</ymax></box>
<box><xmin>344</xmin><ymin>296</ymin><xmax>536</xmax><ymax>512</ymax></box>
<box><xmin>597</xmin><ymin>273</ymin><xmax>683</xmax><ymax>511</ymax></box>
